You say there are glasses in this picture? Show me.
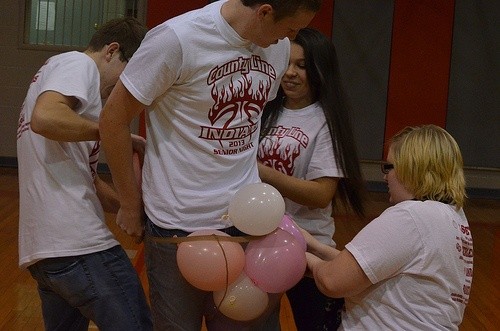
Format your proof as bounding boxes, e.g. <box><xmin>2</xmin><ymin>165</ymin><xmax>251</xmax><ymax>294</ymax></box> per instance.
<box><xmin>381</xmin><ymin>163</ymin><xmax>393</xmax><ymax>174</ymax></box>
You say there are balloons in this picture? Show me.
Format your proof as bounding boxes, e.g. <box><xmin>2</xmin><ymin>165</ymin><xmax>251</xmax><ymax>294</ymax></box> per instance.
<box><xmin>228</xmin><ymin>181</ymin><xmax>286</xmax><ymax>237</ymax></box>
<box><xmin>281</xmin><ymin>213</ymin><xmax>307</xmax><ymax>246</ymax></box>
<box><xmin>241</xmin><ymin>229</ymin><xmax>307</xmax><ymax>295</ymax></box>
<box><xmin>177</xmin><ymin>228</ymin><xmax>246</xmax><ymax>292</ymax></box>
<box><xmin>214</xmin><ymin>271</ymin><xmax>268</xmax><ymax>321</ymax></box>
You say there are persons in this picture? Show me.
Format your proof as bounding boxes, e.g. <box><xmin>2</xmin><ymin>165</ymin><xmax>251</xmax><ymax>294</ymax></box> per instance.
<box><xmin>17</xmin><ymin>15</ymin><xmax>150</xmax><ymax>331</ymax></box>
<box><xmin>97</xmin><ymin>0</ymin><xmax>326</xmax><ymax>331</ymax></box>
<box><xmin>256</xmin><ymin>27</ymin><xmax>367</xmax><ymax>331</ymax></box>
<box><xmin>291</xmin><ymin>124</ymin><xmax>474</xmax><ymax>331</ymax></box>
